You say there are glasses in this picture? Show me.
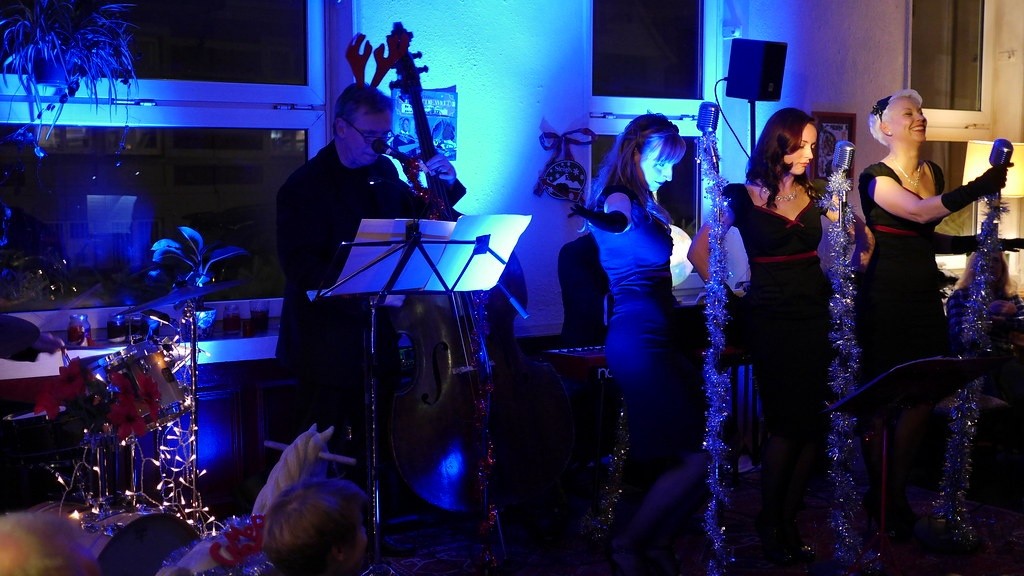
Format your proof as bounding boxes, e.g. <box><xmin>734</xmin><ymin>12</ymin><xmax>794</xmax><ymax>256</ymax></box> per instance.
<box><xmin>343</xmin><ymin>118</ymin><xmax>397</xmax><ymax>145</ymax></box>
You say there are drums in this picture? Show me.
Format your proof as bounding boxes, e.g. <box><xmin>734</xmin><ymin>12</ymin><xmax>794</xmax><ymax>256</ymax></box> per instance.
<box><xmin>25</xmin><ymin>501</ymin><xmax>203</xmax><ymax>576</ymax></box>
<box><xmin>77</xmin><ymin>336</ymin><xmax>189</xmax><ymax>445</ymax></box>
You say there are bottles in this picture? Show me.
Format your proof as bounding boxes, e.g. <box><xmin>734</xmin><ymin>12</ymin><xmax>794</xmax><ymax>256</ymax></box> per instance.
<box><xmin>68</xmin><ymin>314</ymin><xmax>90</xmax><ymax>345</ymax></box>
<box><xmin>223</xmin><ymin>305</ymin><xmax>241</xmax><ymax>333</ymax></box>
<box><xmin>107</xmin><ymin>312</ymin><xmax>158</xmax><ymax>344</ymax></box>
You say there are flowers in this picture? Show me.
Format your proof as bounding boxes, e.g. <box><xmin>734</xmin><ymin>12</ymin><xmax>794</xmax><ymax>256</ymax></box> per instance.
<box><xmin>31</xmin><ymin>355</ymin><xmax>178</xmax><ymax>439</ymax></box>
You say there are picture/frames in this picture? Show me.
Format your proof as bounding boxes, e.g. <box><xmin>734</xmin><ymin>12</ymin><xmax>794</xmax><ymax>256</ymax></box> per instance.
<box><xmin>810</xmin><ymin>110</ymin><xmax>858</xmax><ymax>192</ymax></box>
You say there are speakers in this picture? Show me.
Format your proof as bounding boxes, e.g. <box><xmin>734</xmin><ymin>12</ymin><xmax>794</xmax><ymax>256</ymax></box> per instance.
<box><xmin>725</xmin><ymin>38</ymin><xmax>788</xmax><ymax>102</ymax></box>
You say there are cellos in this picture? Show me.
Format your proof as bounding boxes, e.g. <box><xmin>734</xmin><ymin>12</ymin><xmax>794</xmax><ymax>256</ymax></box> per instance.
<box><xmin>387</xmin><ymin>22</ymin><xmax>579</xmax><ymax>556</ymax></box>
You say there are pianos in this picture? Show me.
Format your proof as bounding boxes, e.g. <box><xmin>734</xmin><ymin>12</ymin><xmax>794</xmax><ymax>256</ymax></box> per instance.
<box><xmin>541</xmin><ymin>345</ymin><xmax>609</xmax><ymax>385</ymax></box>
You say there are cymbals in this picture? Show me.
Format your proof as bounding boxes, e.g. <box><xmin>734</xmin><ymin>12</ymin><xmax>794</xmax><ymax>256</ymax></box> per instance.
<box><xmin>114</xmin><ymin>276</ymin><xmax>248</xmax><ymax>318</ymax></box>
<box><xmin>0</xmin><ymin>313</ymin><xmax>42</xmax><ymax>355</ymax></box>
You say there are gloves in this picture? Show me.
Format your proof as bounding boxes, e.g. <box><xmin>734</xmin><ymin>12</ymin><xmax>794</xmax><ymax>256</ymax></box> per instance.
<box><xmin>941</xmin><ymin>164</ymin><xmax>1006</xmax><ymax>212</ymax></box>
<box><xmin>952</xmin><ymin>235</ymin><xmax>1024</xmax><ymax>257</ymax></box>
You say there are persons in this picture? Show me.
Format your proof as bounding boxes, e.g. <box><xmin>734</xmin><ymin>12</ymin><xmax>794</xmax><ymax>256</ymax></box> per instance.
<box><xmin>271</xmin><ymin>83</ymin><xmax>466</xmax><ymax>497</ymax></box>
<box><xmin>262</xmin><ymin>477</ymin><xmax>367</xmax><ymax>576</ymax></box>
<box><xmin>566</xmin><ymin>88</ymin><xmax>1024</xmax><ymax>575</ymax></box>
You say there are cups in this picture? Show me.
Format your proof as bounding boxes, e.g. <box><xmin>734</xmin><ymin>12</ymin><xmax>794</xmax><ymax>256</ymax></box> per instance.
<box><xmin>249</xmin><ymin>300</ymin><xmax>271</xmax><ymax>330</ymax></box>
<box><xmin>244</xmin><ymin>320</ymin><xmax>254</xmax><ymax>337</ymax></box>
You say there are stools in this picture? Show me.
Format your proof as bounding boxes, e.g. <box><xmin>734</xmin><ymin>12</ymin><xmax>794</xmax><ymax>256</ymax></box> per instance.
<box><xmin>543</xmin><ymin>344</ymin><xmax>771</xmax><ymax>566</ymax></box>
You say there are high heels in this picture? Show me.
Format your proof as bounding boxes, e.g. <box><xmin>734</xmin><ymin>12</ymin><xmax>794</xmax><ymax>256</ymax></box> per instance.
<box><xmin>641</xmin><ymin>543</ymin><xmax>686</xmax><ymax>576</ymax></box>
<box><xmin>605</xmin><ymin>535</ymin><xmax>656</xmax><ymax>576</ymax></box>
<box><xmin>755</xmin><ymin>516</ymin><xmax>815</xmax><ymax>567</ymax></box>
<box><xmin>863</xmin><ymin>488</ymin><xmax>919</xmax><ymax>541</ymax></box>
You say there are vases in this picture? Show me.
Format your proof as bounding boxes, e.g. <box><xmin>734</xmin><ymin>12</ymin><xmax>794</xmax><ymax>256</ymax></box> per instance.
<box><xmin>80</xmin><ymin>420</ymin><xmax>139</xmax><ymax>543</ymax></box>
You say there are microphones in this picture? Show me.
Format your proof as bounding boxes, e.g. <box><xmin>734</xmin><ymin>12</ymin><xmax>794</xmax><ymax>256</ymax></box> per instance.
<box><xmin>371</xmin><ymin>139</ymin><xmax>419</xmax><ymax>169</ymax></box>
<box><xmin>989</xmin><ymin>139</ymin><xmax>1014</xmax><ymax>167</ymax></box>
<box><xmin>832</xmin><ymin>140</ymin><xmax>855</xmax><ymax>212</ymax></box>
<box><xmin>696</xmin><ymin>101</ymin><xmax>720</xmax><ymax>173</ymax></box>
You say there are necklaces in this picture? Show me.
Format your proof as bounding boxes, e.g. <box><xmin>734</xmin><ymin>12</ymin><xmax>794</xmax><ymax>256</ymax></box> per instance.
<box><xmin>889</xmin><ymin>156</ymin><xmax>921</xmax><ymax>188</ymax></box>
<box><xmin>758</xmin><ymin>180</ymin><xmax>799</xmax><ymax>201</ymax></box>
